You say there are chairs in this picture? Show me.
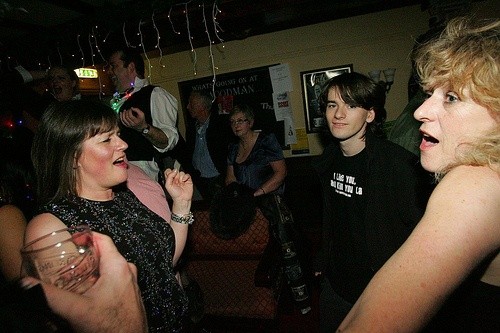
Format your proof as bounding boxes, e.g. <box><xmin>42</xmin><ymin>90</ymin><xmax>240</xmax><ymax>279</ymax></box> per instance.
<box><xmin>181</xmin><ymin>190</ymin><xmax>284</xmax><ymax>333</ymax></box>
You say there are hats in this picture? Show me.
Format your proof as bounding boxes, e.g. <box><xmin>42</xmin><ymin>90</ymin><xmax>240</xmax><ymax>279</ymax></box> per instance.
<box><xmin>208</xmin><ymin>182</ymin><xmax>256</xmax><ymax>240</ymax></box>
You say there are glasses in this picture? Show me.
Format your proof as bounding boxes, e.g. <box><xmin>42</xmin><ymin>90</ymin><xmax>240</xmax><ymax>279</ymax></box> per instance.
<box><xmin>231</xmin><ymin>118</ymin><xmax>249</xmax><ymax>124</ymax></box>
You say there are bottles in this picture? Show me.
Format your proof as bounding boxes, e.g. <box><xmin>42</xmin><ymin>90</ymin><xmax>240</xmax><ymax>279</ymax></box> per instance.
<box><xmin>24</xmin><ymin>183</ymin><xmax>32</xmax><ymax>200</ymax></box>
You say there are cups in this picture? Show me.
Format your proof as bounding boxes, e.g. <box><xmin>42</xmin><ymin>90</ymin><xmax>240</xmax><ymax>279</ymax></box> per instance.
<box><xmin>20</xmin><ymin>225</ymin><xmax>100</xmax><ymax>294</ymax></box>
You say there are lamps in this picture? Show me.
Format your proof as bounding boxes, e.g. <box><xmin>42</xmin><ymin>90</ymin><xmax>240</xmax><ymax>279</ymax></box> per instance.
<box><xmin>369</xmin><ymin>67</ymin><xmax>397</xmax><ymax>94</ymax></box>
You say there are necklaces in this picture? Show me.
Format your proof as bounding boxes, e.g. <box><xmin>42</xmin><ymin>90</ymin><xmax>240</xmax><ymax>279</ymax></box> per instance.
<box><xmin>114</xmin><ymin>84</ymin><xmax>136</xmax><ymax>103</ymax></box>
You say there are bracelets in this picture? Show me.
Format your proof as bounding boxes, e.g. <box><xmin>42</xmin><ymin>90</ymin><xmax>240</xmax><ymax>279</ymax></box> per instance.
<box><xmin>260</xmin><ymin>186</ymin><xmax>266</xmax><ymax>195</ymax></box>
<box><xmin>170</xmin><ymin>210</ymin><xmax>194</xmax><ymax>225</ymax></box>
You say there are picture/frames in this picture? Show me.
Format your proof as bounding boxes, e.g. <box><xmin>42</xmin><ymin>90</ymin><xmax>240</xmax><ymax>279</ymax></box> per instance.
<box><xmin>300</xmin><ymin>64</ymin><xmax>354</xmax><ymax>134</ymax></box>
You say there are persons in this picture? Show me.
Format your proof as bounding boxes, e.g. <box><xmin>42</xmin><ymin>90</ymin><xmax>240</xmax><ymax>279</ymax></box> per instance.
<box><xmin>29</xmin><ymin>93</ymin><xmax>195</xmax><ymax>333</ymax></box>
<box><xmin>224</xmin><ymin>104</ymin><xmax>289</xmax><ymax>248</ymax></box>
<box><xmin>0</xmin><ymin>230</ymin><xmax>151</xmax><ymax>333</ymax></box>
<box><xmin>388</xmin><ymin>27</ymin><xmax>446</xmax><ymax>160</ymax></box>
<box><xmin>184</xmin><ymin>90</ymin><xmax>236</xmax><ymax>205</ymax></box>
<box><xmin>47</xmin><ymin>63</ymin><xmax>83</xmax><ymax>102</ymax></box>
<box><xmin>105</xmin><ymin>46</ymin><xmax>184</xmax><ymax>211</ymax></box>
<box><xmin>318</xmin><ymin>72</ymin><xmax>441</xmax><ymax>307</ymax></box>
<box><xmin>307</xmin><ymin>72</ymin><xmax>332</xmax><ymax>120</ymax></box>
<box><xmin>1</xmin><ymin>128</ymin><xmax>30</xmax><ymax>284</ymax></box>
<box><xmin>335</xmin><ymin>8</ymin><xmax>500</xmax><ymax>333</ymax></box>
<box><xmin>120</xmin><ymin>149</ymin><xmax>172</xmax><ymax>224</ymax></box>
